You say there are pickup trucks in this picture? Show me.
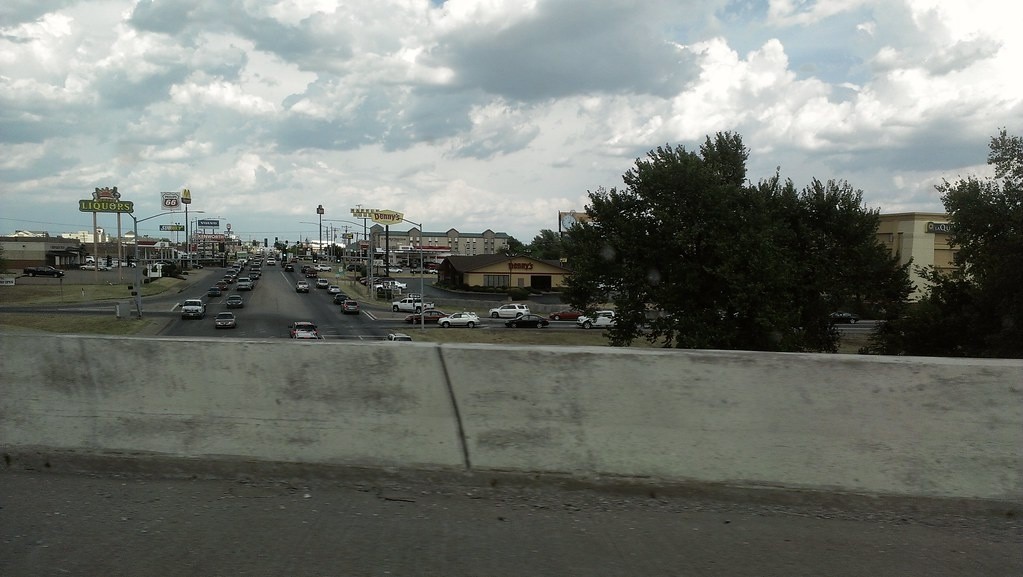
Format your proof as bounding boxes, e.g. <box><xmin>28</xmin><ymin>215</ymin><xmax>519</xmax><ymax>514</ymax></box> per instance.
<box><xmin>178</xmin><ymin>299</ymin><xmax>206</xmax><ymax>320</ymax></box>
<box><xmin>576</xmin><ymin>310</ymin><xmax>630</xmax><ymax>329</ymax></box>
<box><xmin>287</xmin><ymin>320</ymin><xmax>318</xmax><ymax>339</ymax></box>
<box><xmin>391</xmin><ymin>293</ymin><xmax>435</xmax><ymax>313</ymax></box>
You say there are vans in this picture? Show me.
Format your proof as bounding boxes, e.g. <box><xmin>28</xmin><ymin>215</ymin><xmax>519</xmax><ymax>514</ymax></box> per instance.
<box><xmin>23</xmin><ymin>264</ymin><xmax>65</xmax><ymax>277</ymax></box>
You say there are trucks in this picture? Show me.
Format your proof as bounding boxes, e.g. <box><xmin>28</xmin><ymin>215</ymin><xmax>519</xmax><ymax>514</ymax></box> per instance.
<box><xmin>383</xmin><ymin>333</ymin><xmax>413</xmax><ymax>342</ymax></box>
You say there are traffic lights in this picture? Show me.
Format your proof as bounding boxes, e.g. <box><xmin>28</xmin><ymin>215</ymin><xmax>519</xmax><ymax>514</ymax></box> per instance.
<box><xmin>107</xmin><ymin>255</ymin><xmax>113</xmax><ymax>266</ymax></box>
<box><xmin>336</xmin><ymin>251</ymin><xmax>341</xmax><ymax>263</ymax></box>
<box><xmin>313</xmin><ymin>253</ymin><xmax>317</xmax><ymax>262</ymax></box>
<box><xmin>282</xmin><ymin>247</ymin><xmax>287</xmax><ymax>257</ymax></box>
<box><xmin>127</xmin><ymin>255</ymin><xmax>134</xmax><ymax>268</ymax></box>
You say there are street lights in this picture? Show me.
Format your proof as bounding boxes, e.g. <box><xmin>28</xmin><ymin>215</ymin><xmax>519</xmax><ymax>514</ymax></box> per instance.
<box><xmin>134</xmin><ymin>209</ymin><xmax>206</xmax><ymax>310</ymax></box>
<box><xmin>322</xmin><ymin>218</ymin><xmax>373</xmax><ymax>299</ymax></box>
<box><xmin>299</xmin><ymin>222</ymin><xmax>329</xmax><ymax>261</ymax></box>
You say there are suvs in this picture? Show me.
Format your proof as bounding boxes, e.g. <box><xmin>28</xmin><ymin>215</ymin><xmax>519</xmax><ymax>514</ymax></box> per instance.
<box><xmin>489</xmin><ymin>304</ymin><xmax>530</xmax><ymax>318</ymax></box>
<box><xmin>347</xmin><ymin>264</ymin><xmax>362</xmax><ymax>272</ymax></box>
<box><xmin>314</xmin><ymin>264</ymin><xmax>332</xmax><ymax>272</ymax></box>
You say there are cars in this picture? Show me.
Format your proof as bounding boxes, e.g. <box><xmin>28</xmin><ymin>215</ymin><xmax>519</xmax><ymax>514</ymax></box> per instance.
<box><xmin>828</xmin><ymin>311</ymin><xmax>861</xmax><ymax>325</ymax></box>
<box><xmin>404</xmin><ymin>310</ymin><xmax>482</xmax><ymax>329</ymax></box>
<box><xmin>505</xmin><ymin>314</ymin><xmax>549</xmax><ymax>329</ymax></box>
<box><xmin>207</xmin><ymin>251</ymin><xmax>264</xmax><ymax>297</ymax></box>
<box><xmin>192</xmin><ymin>263</ymin><xmax>203</xmax><ymax>269</ymax></box>
<box><xmin>410</xmin><ymin>266</ymin><xmax>439</xmax><ymax>275</ymax></box>
<box><xmin>384</xmin><ymin>266</ymin><xmax>403</xmax><ymax>274</ymax></box>
<box><xmin>285</xmin><ymin>264</ymin><xmax>359</xmax><ymax>315</ymax></box>
<box><xmin>214</xmin><ymin>312</ymin><xmax>236</xmax><ymax>328</ymax></box>
<box><xmin>549</xmin><ymin>306</ymin><xmax>583</xmax><ymax>321</ymax></box>
<box><xmin>226</xmin><ymin>295</ymin><xmax>244</xmax><ymax>308</ymax></box>
<box><xmin>79</xmin><ymin>256</ymin><xmax>136</xmax><ymax>271</ymax></box>
<box><xmin>267</xmin><ymin>258</ymin><xmax>275</xmax><ymax>265</ymax></box>
<box><xmin>359</xmin><ymin>276</ymin><xmax>407</xmax><ymax>291</ymax></box>
